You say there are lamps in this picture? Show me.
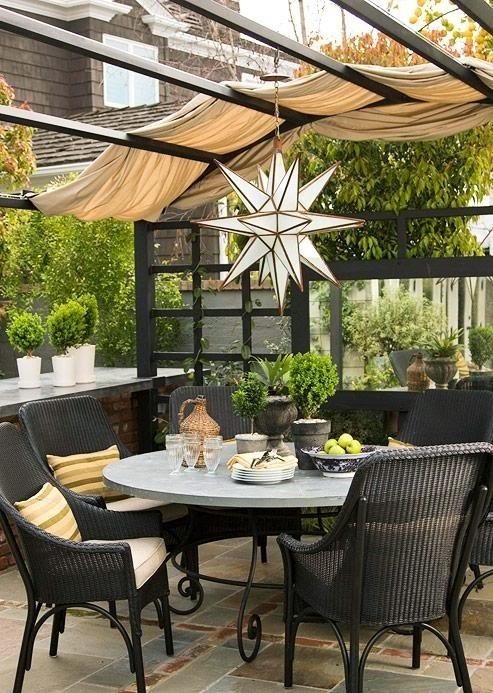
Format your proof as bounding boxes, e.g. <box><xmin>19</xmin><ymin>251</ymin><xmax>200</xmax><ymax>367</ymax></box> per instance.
<box><xmin>186</xmin><ymin>39</ymin><xmax>368</xmax><ymax>318</ymax></box>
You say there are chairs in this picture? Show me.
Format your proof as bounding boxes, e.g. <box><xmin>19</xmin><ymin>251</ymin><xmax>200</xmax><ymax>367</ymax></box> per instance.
<box><xmin>160</xmin><ymin>381</ymin><xmax>306</xmax><ymax>604</ymax></box>
<box><xmin>455</xmin><ymin>373</ymin><xmax>491</xmax><ymax>593</ymax></box>
<box><xmin>17</xmin><ymin>389</ymin><xmax>207</xmax><ymax>631</ymax></box>
<box><xmin>0</xmin><ymin>415</ymin><xmax>180</xmax><ymax>693</ymax></box>
<box><xmin>266</xmin><ymin>436</ymin><xmax>492</xmax><ymax>693</ymax></box>
<box><xmin>394</xmin><ymin>387</ymin><xmax>492</xmax><ymax>449</ymax></box>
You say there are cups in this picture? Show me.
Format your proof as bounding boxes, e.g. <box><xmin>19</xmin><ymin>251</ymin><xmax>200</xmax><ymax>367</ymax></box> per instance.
<box><xmin>182</xmin><ymin>433</ymin><xmax>200</xmax><ymax>471</ymax></box>
<box><xmin>165</xmin><ymin>433</ymin><xmax>183</xmax><ymax>475</ymax></box>
<box><xmin>202</xmin><ymin>435</ymin><xmax>223</xmax><ymax>472</ymax></box>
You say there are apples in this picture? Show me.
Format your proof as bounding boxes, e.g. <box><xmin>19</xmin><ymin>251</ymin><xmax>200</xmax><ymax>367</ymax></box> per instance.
<box><xmin>318</xmin><ymin>433</ymin><xmax>362</xmax><ymax>455</ymax></box>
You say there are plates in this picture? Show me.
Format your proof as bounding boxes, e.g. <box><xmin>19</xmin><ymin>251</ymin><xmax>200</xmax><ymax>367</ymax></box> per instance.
<box><xmin>231</xmin><ymin>459</ymin><xmax>296</xmax><ymax>484</ymax></box>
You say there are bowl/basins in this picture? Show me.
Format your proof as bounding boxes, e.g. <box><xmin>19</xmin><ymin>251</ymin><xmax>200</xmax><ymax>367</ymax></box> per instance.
<box><xmin>299</xmin><ymin>445</ymin><xmax>377</xmax><ymax>476</ymax></box>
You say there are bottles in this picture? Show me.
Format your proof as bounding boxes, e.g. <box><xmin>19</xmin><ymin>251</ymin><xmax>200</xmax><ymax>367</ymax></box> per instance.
<box><xmin>407</xmin><ymin>352</ymin><xmax>431</xmax><ymax>390</ymax></box>
<box><xmin>177</xmin><ymin>394</ymin><xmax>220</xmax><ymax>467</ymax></box>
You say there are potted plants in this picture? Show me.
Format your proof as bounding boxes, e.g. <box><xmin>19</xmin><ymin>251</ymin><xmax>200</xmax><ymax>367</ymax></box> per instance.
<box><xmin>364</xmin><ymin>292</ymin><xmax>447</xmax><ymax>385</ymax></box>
<box><xmin>424</xmin><ymin>325</ymin><xmax>464</xmax><ymax>389</ymax></box>
<box><xmin>246</xmin><ymin>351</ymin><xmax>304</xmax><ymax>452</ymax></box>
<box><xmin>466</xmin><ymin>324</ymin><xmax>492</xmax><ymax>379</ymax></box>
<box><xmin>6</xmin><ymin>308</ymin><xmax>50</xmax><ymax>392</ymax></box>
<box><xmin>283</xmin><ymin>348</ymin><xmax>341</xmax><ymax>473</ymax></box>
<box><xmin>74</xmin><ymin>293</ymin><xmax>99</xmax><ymax>384</ymax></box>
<box><xmin>231</xmin><ymin>366</ymin><xmax>271</xmax><ymax>454</ymax></box>
<box><xmin>45</xmin><ymin>295</ymin><xmax>89</xmax><ymax>391</ymax></box>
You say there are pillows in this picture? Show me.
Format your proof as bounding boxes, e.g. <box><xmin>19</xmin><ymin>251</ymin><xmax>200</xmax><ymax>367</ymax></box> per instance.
<box><xmin>43</xmin><ymin>445</ymin><xmax>135</xmax><ymax>504</ymax></box>
<box><xmin>10</xmin><ymin>477</ymin><xmax>87</xmax><ymax>544</ymax></box>
